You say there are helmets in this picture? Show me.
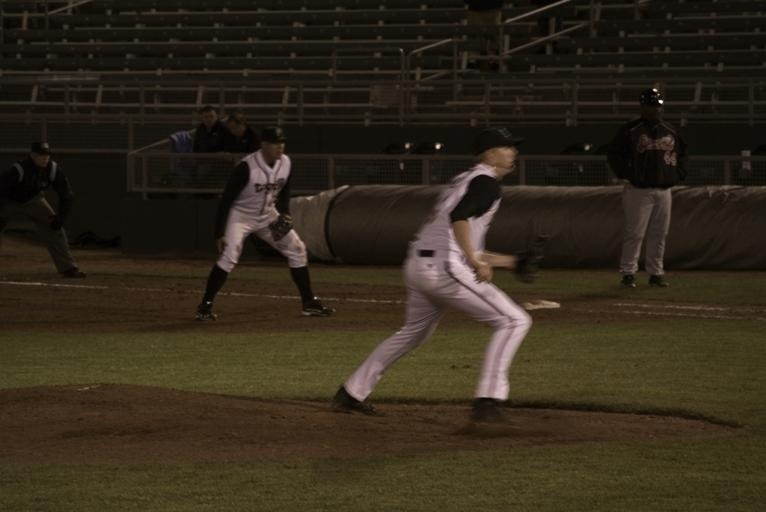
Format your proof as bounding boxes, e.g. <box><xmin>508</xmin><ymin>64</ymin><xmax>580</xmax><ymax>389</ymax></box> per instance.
<box><xmin>639</xmin><ymin>88</ymin><xmax>665</xmax><ymax>107</ymax></box>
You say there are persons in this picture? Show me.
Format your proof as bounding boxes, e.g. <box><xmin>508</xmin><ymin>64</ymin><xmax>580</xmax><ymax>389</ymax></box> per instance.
<box><xmin>330</xmin><ymin>129</ymin><xmax>536</xmax><ymax>438</ymax></box>
<box><xmin>192</xmin><ymin>127</ymin><xmax>336</xmax><ymax>320</ymax></box>
<box><xmin>1</xmin><ymin>139</ymin><xmax>84</xmax><ymax>278</ymax></box>
<box><xmin>192</xmin><ymin>106</ymin><xmax>235</xmax><ymax>201</ymax></box>
<box><xmin>223</xmin><ymin>113</ymin><xmax>262</xmax><ymax>152</ymax></box>
<box><xmin>460</xmin><ymin>0</ymin><xmax>504</xmax><ymax>69</ymax></box>
<box><xmin>608</xmin><ymin>87</ymin><xmax>688</xmax><ymax>288</ymax></box>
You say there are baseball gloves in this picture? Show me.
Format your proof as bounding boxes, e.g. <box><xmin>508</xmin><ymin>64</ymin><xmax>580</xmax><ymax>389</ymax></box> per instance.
<box><xmin>512</xmin><ymin>232</ymin><xmax>552</xmax><ymax>282</ymax></box>
<box><xmin>269</xmin><ymin>212</ymin><xmax>294</xmax><ymax>241</ymax></box>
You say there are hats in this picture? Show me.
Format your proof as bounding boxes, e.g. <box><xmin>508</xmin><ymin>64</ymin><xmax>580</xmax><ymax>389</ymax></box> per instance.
<box><xmin>261</xmin><ymin>128</ymin><xmax>288</xmax><ymax>143</ymax></box>
<box><xmin>470</xmin><ymin>128</ymin><xmax>526</xmax><ymax>155</ymax></box>
<box><xmin>32</xmin><ymin>141</ymin><xmax>53</xmax><ymax>155</ymax></box>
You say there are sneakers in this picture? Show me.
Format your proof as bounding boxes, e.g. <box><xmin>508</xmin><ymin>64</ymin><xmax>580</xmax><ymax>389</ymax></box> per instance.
<box><xmin>649</xmin><ymin>275</ymin><xmax>670</xmax><ymax>288</ymax></box>
<box><xmin>302</xmin><ymin>297</ymin><xmax>335</xmax><ymax>317</ymax></box>
<box><xmin>62</xmin><ymin>267</ymin><xmax>86</xmax><ymax>278</ymax></box>
<box><xmin>196</xmin><ymin>301</ymin><xmax>214</xmax><ymax>320</ymax></box>
<box><xmin>621</xmin><ymin>275</ymin><xmax>637</xmax><ymax>288</ymax></box>
<box><xmin>474</xmin><ymin>399</ymin><xmax>510</xmax><ymax>424</ymax></box>
<box><xmin>334</xmin><ymin>386</ymin><xmax>383</xmax><ymax>416</ymax></box>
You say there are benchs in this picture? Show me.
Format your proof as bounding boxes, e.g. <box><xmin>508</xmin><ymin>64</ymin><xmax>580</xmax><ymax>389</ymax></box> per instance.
<box><xmin>0</xmin><ymin>0</ymin><xmax>766</xmax><ymax>201</ymax></box>
